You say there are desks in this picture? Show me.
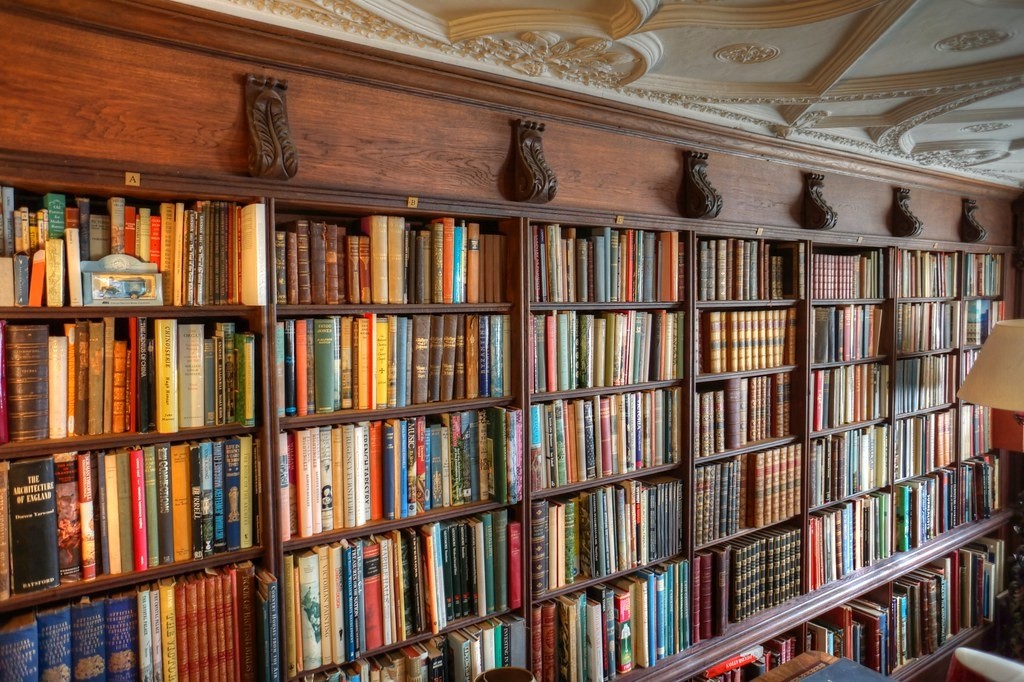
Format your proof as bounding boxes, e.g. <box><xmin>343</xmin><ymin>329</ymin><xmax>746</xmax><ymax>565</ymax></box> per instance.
<box><xmin>747</xmin><ymin>650</ymin><xmax>898</xmax><ymax>682</ymax></box>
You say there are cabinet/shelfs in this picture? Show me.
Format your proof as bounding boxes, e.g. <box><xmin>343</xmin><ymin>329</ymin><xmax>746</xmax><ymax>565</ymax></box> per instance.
<box><xmin>0</xmin><ymin>0</ymin><xmax>1024</xmax><ymax>682</ymax></box>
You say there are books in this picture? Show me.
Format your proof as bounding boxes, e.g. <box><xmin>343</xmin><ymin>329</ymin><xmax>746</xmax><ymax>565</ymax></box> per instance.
<box><xmin>0</xmin><ymin>183</ymin><xmax>1005</xmax><ymax>682</ymax></box>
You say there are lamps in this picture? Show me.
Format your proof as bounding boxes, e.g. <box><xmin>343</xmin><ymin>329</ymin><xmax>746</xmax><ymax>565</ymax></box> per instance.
<box><xmin>956</xmin><ymin>319</ymin><xmax>1024</xmax><ymax>412</ymax></box>
